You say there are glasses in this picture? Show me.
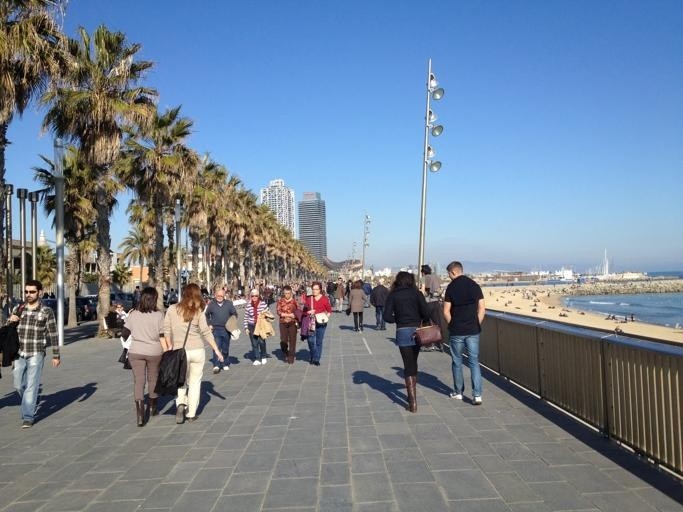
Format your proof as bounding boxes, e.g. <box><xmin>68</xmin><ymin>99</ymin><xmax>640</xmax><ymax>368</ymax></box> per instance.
<box><xmin>25</xmin><ymin>290</ymin><xmax>36</xmax><ymax>294</ymax></box>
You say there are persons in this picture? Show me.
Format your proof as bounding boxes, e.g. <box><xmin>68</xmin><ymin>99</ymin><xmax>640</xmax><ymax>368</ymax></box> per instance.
<box><xmin>275</xmin><ymin>285</ymin><xmax>298</xmax><ymax>365</ymax></box>
<box><xmin>243</xmin><ymin>288</ymin><xmax>270</xmax><ymax>367</ymax></box>
<box><xmin>164</xmin><ymin>283</ymin><xmax>225</xmax><ymax>424</ymax></box>
<box><xmin>344</xmin><ymin>279</ymin><xmax>352</xmax><ymax>298</ymax></box>
<box><xmin>370</xmin><ymin>278</ymin><xmax>389</xmax><ymax>330</ymax></box>
<box><xmin>332</xmin><ymin>278</ymin><xmax>346</xmax><ymax>313</ymax></box>
<box><xmin>381</xmin><ymin>271</ymin><xmax>431</xmax><ymax>412</ymax></box>
<box><xmin>121</xmin><ymin>286</ymin><xmax>164</xmax><ymax>427</ymax></box>
<box><xmin>442</xmin><ymin>260</ymin><xmax>486</xmax><ymax>405</ymax></box>
<box><xmin>8</xmin><ymin>279</ymin><xmax>61</xmax><ymax>430</ymax></box>
<box><xmin>302</xmin><ymin>281</ymin><xmax>332</xmax><ymax>367</ymax></box>
<box><xmin>348</xmin><ymin>281</ymin><xmax>368</xmax><ymax>332</ymax></box>
<box><xmin>419</xmin><ymin>264</ymin><xmax>444</xmax><ymax>353</ymax></box>
<box><xmin>205</xmin><ymin>289</ymin><xmax>238</xmax><ymax>374</ymax></box>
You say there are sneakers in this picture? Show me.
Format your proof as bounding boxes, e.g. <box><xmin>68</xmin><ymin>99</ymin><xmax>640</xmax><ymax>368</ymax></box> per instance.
<box><xmin>253</xmin><ymin>360</ymin><xmax>261</xmax><ymax>365</ymax></box>
<box><xmin>188</xmin><ymin>418</ymin><xmax>196</xmax><ymax>422</ymax></box>
<box><xmin>22</xmin><ymin>421</ymin><xmax>32</xmax><ymax>428</ymax></box>
<box><xmin>223</xmin><ymin>366</ymin><xmax>229</xmax><ymax>370</ymax></box>
<box><xmin>472</xmin><ymin>395</ymin><xmax>482</xmax><ymax>404</ymax></box>
<box><xmin>213</xmin><ymin>366</ymin><xmax>220</xmax><ymax>373</ymax></box>
<box><xmin>419</xmin><ymin>343</ymin><xmax>434</xmax><ymax>352</ymax></box>
<box><xmin>176</xmin><ymin>404</ymin><xmax>184</xmax><ymax>424</ymax></box>
<box><xmin>262</xmin><ymin>358</ymin><xmax>267</xmax><ymax>365</ymax></box>
<box><xmin>450</xmin><ymin>391</ymin><xmax>463</xmax><ymax>400</ymax></box>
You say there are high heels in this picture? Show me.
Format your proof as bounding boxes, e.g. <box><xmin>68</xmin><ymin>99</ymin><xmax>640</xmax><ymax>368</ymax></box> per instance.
<box><xmin>360</xmin><ymin>323</ymin><xmax>363</xmax><ymax>330</ymax></box>
<box><xmin>353</xmin><ymin>328</ymin><xmax>358</xmax><ymax>332</ymax></box>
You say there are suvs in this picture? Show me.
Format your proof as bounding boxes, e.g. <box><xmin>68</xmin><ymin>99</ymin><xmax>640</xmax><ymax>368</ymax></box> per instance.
<box><xmin>42</xmin><ymin>299</ymin><xmax>82</xmax><ymax>325</ymax></box>
<box><xmin>108</xmin><ymin>293</ymin><xmax>133</xmax><ymax>310</ymax></box>
<box><xmin>76</xmin><ymin>297</ymin><xmax>96</xmax><ymax>320</ymax></box>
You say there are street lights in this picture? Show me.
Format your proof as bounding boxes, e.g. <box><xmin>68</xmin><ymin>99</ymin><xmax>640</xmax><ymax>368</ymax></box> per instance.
<box><xmin>175</xmin><ymin>198</ymin><xmax>182</xmax><ymax>303</ymax></box>
<box><xmin>361</xmin><ymin>215</ymin><xmax>372</xmax><ymax>280</ymax></box>
<box><xmin>417</xmin><ymin>57</ymin><xmax>445</xmax><ymax>289</ymax></box>
<box><xmin>52</xmin><ymin>137</ymin><xmax>66</xmax><ymax>346</ymax></box>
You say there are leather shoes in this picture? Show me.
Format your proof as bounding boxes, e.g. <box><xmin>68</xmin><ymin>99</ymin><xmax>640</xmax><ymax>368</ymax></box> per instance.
<box><xmin>314</xmin><ymin>361</ymin><xmax>320</xmax><ymax>366</ymax></box>
<box><xmin>310</xmin><ymin>360</ymin><xmax>314</xmax><ymax>363</ymax></box>
<box><xmin>382</xmin><ymin>326</ymin><xmax>385</xmax><ymax>330</ymax></box>
<box><xmin>374</xmin><ymin>324</ymin><xmax>380</xmax><ymax>330</ymax></box>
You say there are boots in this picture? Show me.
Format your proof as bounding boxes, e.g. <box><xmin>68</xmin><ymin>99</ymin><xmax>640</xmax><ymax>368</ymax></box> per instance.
<box><xmin>135</xmin><ymin>400</ymin><xmax>145</xmax><ymax>425</ymax></box>
<box><xmin>146</xmin><ymin>396</ymin><xmax>158</xmax><ymax>416</ymax></box>
<box><xmin>406</xmin><ymin>376</ymin><xmax>417</xmax><ymax>402</ymax></box>
<box><xmin>405</xmin><ymin>376</ymin><xmax>416</xmax><ymax>413</ymax></box>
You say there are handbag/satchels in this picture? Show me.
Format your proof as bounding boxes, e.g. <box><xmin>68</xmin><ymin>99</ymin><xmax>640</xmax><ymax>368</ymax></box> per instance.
<box><xmin>310</xmin><ymin>295</ymin><xmax>316</xmax><ymax>332</ymax></box>
<box><xmin>154</xmin><ymin>319</ymin><xmax>192</xmax><ymax>394</ymax></box>
<box><xmin>415</xmin><ymin>316</ymin><xmax>443</xmax><ymax>345</ymax></box>
<box><xmin>315</xmin><ymin>311</ymin><xmax>329</xmax><ymax>325</ymax></box>
